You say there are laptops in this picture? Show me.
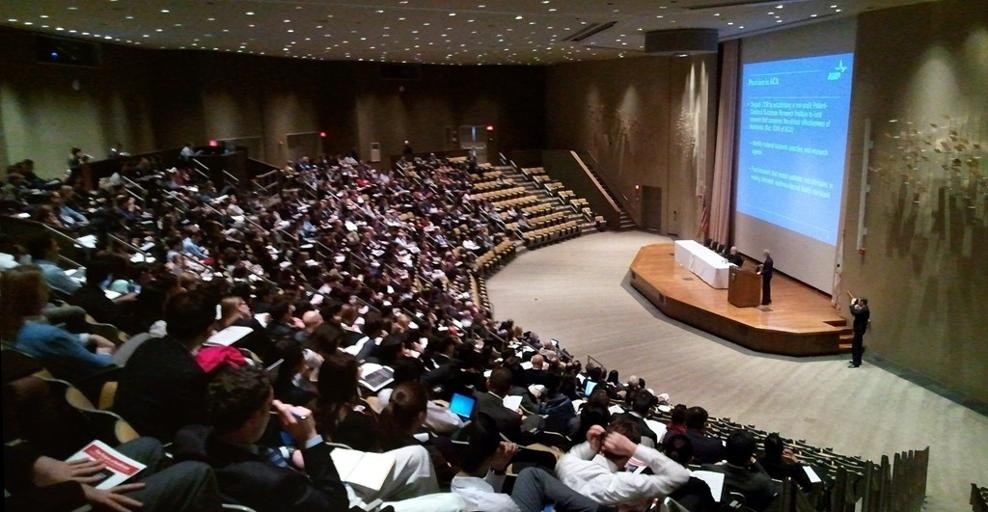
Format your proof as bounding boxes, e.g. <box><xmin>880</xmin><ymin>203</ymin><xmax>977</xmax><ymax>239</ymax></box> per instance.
<box><xmin>583</xmin><ymin>380</ymin><xmax>597</xmax><ymax>397</ymax></box>
<box><xmin>446</xmin><ymin>392</ymin><xmax>478</xmax><ymax>422</ymax></box>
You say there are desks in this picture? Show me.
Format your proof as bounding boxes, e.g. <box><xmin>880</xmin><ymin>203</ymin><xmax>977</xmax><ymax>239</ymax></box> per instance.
<box><xmin>674</xmin><ymin>239</ymin><xmax>738</xmax><ymax>289</ymax></box>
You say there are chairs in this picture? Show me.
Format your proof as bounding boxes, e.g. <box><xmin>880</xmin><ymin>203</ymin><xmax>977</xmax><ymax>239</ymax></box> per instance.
<box><xmin>9</xmin><ymin>314</ymin><xmax>876</xmax><ymax>512</ymax></box>
<box><xmin>410</xmin><ymin>155</ymin><xmax>610</xmax><ymax>310</ymax></box>
<box><xmin>979</xmin><ymin>485</ymin><xmax>988</xmax><ymax>512</ymax></box>
<box><xmin>704</xmin><ymin>239</ymin><xmax>729</xmax><ymax>258</ymax></box>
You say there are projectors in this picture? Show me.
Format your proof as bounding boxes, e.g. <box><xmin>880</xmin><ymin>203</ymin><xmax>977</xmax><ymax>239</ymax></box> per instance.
<box><xmin>45</xmin><ymin>48</ymin><xmax>65</xmax><ymax>63</ymax></box>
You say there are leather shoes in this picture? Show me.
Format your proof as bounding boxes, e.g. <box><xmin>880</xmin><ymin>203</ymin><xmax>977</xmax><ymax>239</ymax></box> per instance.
<box><xmin>849</xmin><ymin>360</ymin><xmax>862</xmax><ymax>368</ymax></box>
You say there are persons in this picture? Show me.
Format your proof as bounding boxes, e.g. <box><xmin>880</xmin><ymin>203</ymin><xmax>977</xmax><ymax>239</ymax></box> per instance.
<box><xmin>0</xmin><ymin>139</ymin><xmax>803</xmax><ymax>512</ymax></box>
<box><xmin>729</xmin><ymin>246</ymin><xmax>742</xmax><ymax>265</ymax></box>
<box><xmin>848</xmin><ymin>296</ymin><xmax>869</xmax><ymax>368</ymax></box>
<box><xmin>757</xmin><ymin>248</ymin><xmax>774</xmax><ymax>305</ymax></box>
